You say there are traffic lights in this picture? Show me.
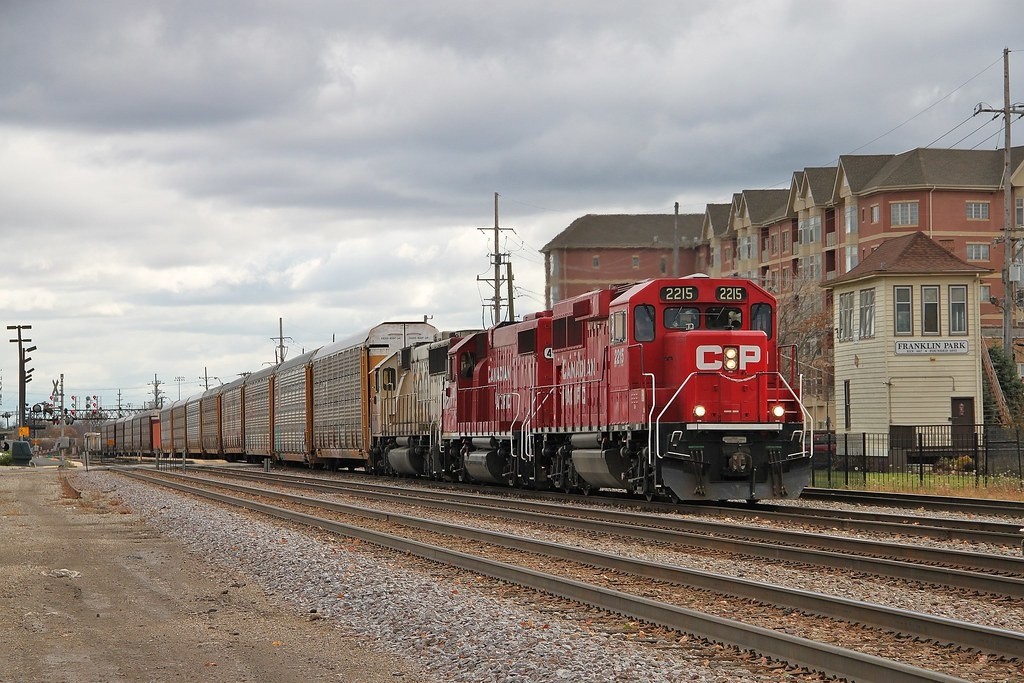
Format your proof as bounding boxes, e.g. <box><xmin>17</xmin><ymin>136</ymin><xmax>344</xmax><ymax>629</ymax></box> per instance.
<box><xmin>56</xmin><ymin>420</ymin><xmax>59</xmax><ymax>424</ymax></box>
<box><xmin>71</xmin><ymin>403</ymin><xmax>75</xmax><ymax>407</ymax></box>
<box><xmin>50</xmin><ymin>396</ymin><xmax>53</xmax><ymax>400</ymax></box>
<box><xmin>93</xmin><ymin>403</ymin><xmax>96</xmax><ymax>407</ymax></box>
<box><xmin>71</xmin><ymin>410</ymin><xmax>75</xmax><ymax>414</ymax></box>
<box><xmin>86</xmin><ymin>396</ymin><xmax>90</xmax><ymax>400</ymax></box>
<box><xmin>93</xmin><ymin>395</ymin><xmax>96</xmax><ymax>399</ymax></box>
<box><xmin>50</xmin><ymin>404</ymin><xmax>53</xmax><ymax>407</ymax></box>
<box><xmin>50</xmin><ymin>410</ymin><xmax>53</xmax><ymax>414</ymax></box>
<box><xmin>85</xmin><ymin>404</ymin><xmax>91</xmax><ymax>408</ymax></box>
<box><xmin>93</xmin><ymin>409</ymin><xmax>96</xmax><ymax>414</ymax></box>
<box><xmin>71</xmin><ymin>395</ymin><xmax>75</xmax><ymax>399</ymax></box>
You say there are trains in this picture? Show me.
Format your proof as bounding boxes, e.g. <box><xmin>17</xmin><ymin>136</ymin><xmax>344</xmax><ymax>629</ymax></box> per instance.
<box><xmin>100</xmin><ymin>274</ymin><xmax>815</xmax><ymax>505</ymax></box>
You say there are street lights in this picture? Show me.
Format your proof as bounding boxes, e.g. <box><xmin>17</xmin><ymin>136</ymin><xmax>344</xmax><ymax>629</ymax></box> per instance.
<box><xmin>989</xmin><ymin>294</ymin><xmax>1014</xmax><ymax>364</ymax></box>
<box><xmin>22</xmin><ymin>345</ymin><xmax>37</xmax><ymax>438</ymax></box>
<box><xmin>5</xmin><ymin>323</ymin><xmax>33</xmax><ymax>440</ymax></box>
